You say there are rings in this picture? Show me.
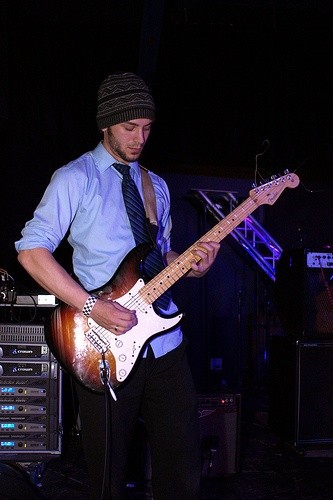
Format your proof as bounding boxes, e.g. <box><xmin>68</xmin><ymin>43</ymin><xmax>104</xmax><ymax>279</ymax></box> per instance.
<box><xmin>114</xmin><ymin>325</ymin><xmax>120</xmax><ymax>332</ymax></box>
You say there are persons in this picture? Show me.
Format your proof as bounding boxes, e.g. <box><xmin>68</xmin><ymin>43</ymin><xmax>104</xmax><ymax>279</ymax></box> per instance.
<box><xmin>16</xmin><ymin>73</ymin><xmax>221</xmax><ymax>499</ymax></box>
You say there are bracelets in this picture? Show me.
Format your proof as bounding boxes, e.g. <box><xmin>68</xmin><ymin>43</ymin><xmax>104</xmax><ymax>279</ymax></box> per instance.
<box><xmin>81</xmin><ymin>294</ymin><xmax>99</xmax><ymax>318</ymax></box>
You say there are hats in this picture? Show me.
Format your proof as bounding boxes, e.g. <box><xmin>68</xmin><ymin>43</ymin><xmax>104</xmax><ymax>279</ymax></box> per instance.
<box><xmin>96</xmin><ymin>72</ymin><xmax>155</xmax><ymax>130</ymax></box>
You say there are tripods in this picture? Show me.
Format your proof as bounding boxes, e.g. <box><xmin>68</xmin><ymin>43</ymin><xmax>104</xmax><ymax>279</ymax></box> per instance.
<box><xmin>240</xmin><ymin>267</ymin><xmax>305</xmax><ymax>458</ymax></box>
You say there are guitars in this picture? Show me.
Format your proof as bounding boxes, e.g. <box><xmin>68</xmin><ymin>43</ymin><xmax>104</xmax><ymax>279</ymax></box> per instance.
<box><xmin>44</xmin><ymin>170</ymin><xmax>299</xmax><ymax>393</ymax></box>
<box><xmin>314</xmin><ymin>256</ymin><xmax>333</xmax><ymax>336</ymax></box>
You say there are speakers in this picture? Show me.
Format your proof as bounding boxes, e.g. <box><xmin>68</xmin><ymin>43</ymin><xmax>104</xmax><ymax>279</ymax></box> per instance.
<box><xmin>273</xmin><ymin>269</ymin><xmax>333</xmax><ymax>340</ymax></box>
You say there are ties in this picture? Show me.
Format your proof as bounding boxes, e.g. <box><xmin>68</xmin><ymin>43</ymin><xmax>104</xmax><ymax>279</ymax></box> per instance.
<box><xmin>112</xmin><ymin>163</ymin><xmax>171</xmax><ymax>311</ymax></box>
